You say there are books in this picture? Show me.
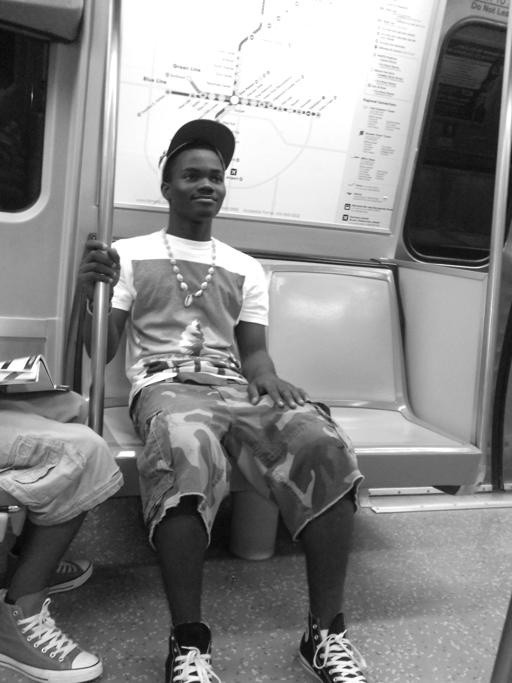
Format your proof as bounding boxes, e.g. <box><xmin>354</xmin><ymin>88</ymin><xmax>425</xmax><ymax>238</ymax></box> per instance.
<box><xmin>0</xmin><ymin>354</ymin><xmax>71</xmax><ymax>394</ymax></box>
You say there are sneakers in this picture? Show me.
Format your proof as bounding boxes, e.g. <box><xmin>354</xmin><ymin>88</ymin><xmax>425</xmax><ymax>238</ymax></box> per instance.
<box><xmin>0</xmin><ymin>549</ymin><xmax>94</xmax><ymax>605</ymax></box>
<box><xmin>0</xmin><ymin>593</ymin><xmax>113</xmax><ymax>683</ymax></box>
<box><xmin>294</xmin><ymin>607</ymin><xmax>371</xmax><ymax>683</ymax></box>
<box><xmin>161</xmin><ymin>616</ymin><xmax>224</xmax><ymax>683</ymax></box>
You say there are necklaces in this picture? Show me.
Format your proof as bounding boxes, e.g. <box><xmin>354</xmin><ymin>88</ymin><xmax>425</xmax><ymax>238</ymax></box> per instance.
<box><xmin>161</xmin><ymin>225</ymin><xmax>217</xmax><ymax>307</ymax></box>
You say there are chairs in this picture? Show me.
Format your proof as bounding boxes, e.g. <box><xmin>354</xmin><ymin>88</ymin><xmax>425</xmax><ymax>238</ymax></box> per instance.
<box><xmin>89</xmin><ymin>260</ymin><xmax>486</xmax><ymax>494</ymax></box>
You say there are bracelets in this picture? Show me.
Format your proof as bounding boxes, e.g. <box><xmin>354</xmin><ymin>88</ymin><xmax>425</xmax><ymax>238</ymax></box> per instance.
<box><xmin>87</xmin><ymin>299</ymin><xmax>113</xmax><ymax>316</ymax></box>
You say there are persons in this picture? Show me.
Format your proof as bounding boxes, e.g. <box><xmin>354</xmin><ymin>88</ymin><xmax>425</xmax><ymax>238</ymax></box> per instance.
<box><xmin>78</xmin><ymin>118</ymin><xmax>372</xmax><ymax>683</ymax></box>
<box><xmin>0</xmin><ymin>389</ymin><xmax>126</xmax><ymax>683</ymax></box>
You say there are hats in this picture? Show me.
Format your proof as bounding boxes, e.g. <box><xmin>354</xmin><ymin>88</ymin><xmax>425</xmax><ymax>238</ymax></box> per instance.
<box><xmin>157</xmin><ymin>118</ymin><xmax>237</xmax><ymax>172</ymax></box>
